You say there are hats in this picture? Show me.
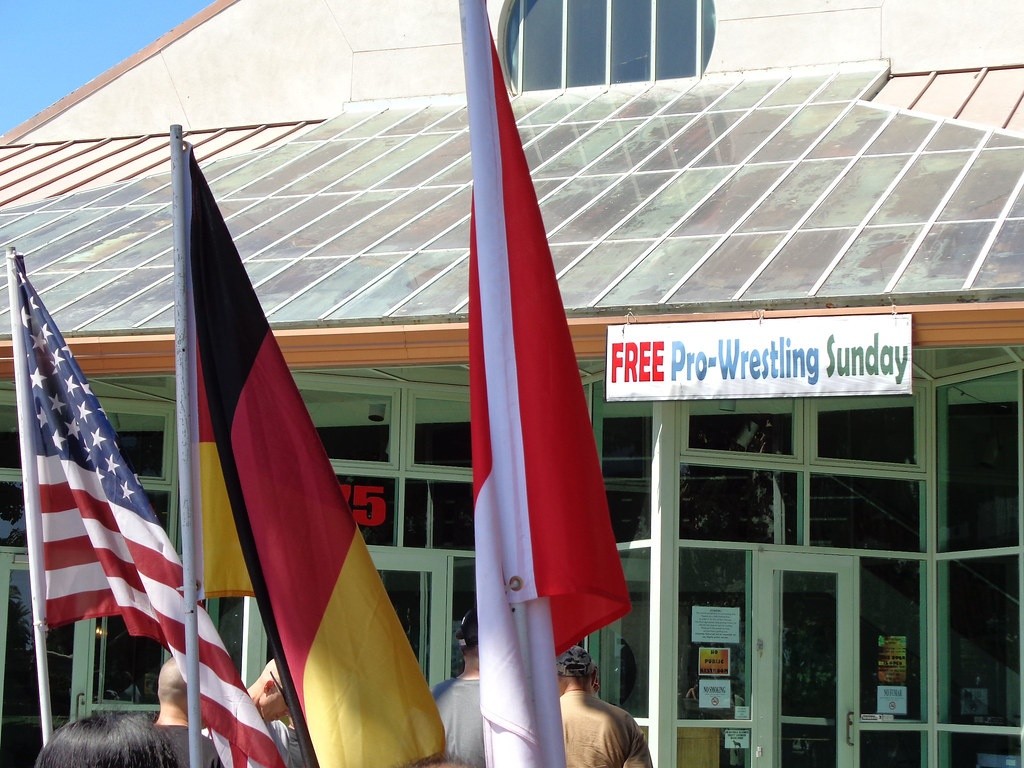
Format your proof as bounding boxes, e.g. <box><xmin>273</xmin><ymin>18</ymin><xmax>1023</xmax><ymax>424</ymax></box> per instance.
<box><xmin>456</xmin><ymin>608</ymin><xmax>478</xmax><ymax>646</ymax></box>
<box><xmin>555</xmin><ymin>645</ymin><xmax>595</xmax><ymax>677</ymax></box>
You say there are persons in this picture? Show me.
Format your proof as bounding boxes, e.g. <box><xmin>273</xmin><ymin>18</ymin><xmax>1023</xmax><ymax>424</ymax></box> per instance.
<box><xmin>556</xmin><ymin>645</ymin><xmax>653</xmax><ymax>768</ymax></box>
<box><xmin>154</xmin><ymin>658</ymin><xmax>306</xmax><ymax>768</ymax></box>
<box><xmin>431</xmin><ymin>607</ymin><xmax>486</xmax><ymax>768</ymax></box>
<box><xmin>35</xmin><ymin>712</ymin><xmax>180</xmax><ymax>768</ymax></box>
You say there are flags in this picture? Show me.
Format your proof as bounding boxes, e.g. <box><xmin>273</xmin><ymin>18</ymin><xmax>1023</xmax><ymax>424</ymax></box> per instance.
<box><xmin>178</xmin><ymin>141</ymin><xmax>446</xmax><ymax>768</ymax></box>
<box><xmin>460</xmin><ymin>0</ymin><xmax>634</xmax><ymax>768</ymax></box>
<box><xmin>6</xmin><ymin>255</ymin><xmax>287</xmax><ymax>768</ymax></box>
